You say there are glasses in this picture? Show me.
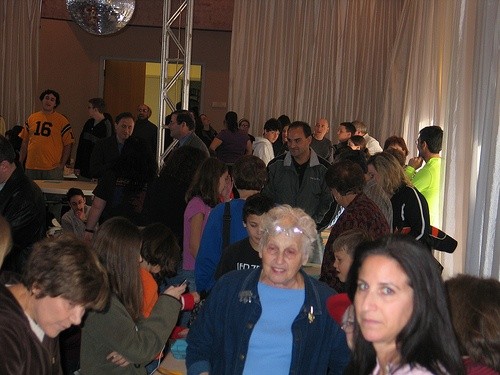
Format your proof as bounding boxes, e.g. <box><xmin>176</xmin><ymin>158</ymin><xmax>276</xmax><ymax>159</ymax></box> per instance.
<box><xmin>266</xmin><ymin>223</ymin><xmax>311</xmax><ymax>242</ymax></box>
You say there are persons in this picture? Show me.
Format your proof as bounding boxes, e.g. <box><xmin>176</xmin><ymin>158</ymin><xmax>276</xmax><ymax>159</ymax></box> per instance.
<box><xmin>0</xmin><ymin>91</ymin><xmax>457</xmax><ymax>375</ymax></box>
<box><xmin>441</xmin><ymin>274</ymin><xmax>500</xmax><ymax>375</ymax></box>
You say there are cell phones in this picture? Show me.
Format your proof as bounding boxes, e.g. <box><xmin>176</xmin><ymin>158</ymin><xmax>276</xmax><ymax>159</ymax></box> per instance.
<box><xmin>179</xmin><ymin>279</ymin><xmax>187</xmax><ymax>287</ymax></box>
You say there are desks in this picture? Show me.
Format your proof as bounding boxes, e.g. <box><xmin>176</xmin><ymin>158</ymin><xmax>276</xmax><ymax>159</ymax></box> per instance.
<box><xmin>31</xmin><ymin>180</ymin><xmax>98</xmax><ymax>225</ymax></box>
